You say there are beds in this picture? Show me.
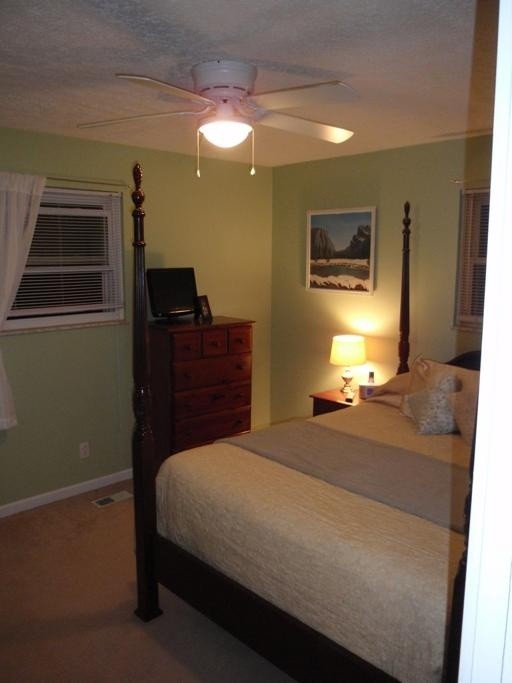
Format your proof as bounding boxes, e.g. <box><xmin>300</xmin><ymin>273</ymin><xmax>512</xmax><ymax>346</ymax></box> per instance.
<box><xmin>130</xmin><ymin>163</ymin><xmax>481</xmax><ymax>683</ymax></box>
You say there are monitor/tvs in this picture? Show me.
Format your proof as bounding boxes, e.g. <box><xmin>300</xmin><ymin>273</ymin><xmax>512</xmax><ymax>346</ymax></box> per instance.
<box><xmin>145</xmin><ymin>267</ymin><xmax>197</xmax><ymax>327</ymax></box>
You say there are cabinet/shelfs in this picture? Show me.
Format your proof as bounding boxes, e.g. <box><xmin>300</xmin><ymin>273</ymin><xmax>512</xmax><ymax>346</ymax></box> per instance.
<box><xmin>143</xmin><ymin>316</ymin><xmax>258</xmax><ymax>457</ymax></box>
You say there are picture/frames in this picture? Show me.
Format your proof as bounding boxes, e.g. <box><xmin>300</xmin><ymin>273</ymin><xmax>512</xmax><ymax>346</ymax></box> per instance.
<box><xmin>306</xmin><ymin>204</ymin><xmax>376</xmax><ymax>296</ymax></box>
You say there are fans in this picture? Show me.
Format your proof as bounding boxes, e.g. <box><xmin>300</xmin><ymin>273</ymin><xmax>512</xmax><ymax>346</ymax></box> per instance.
<box><xmin>78</xmin><ymin>59</ymin><xmax>355</xmax><ymax>144</ymax></box>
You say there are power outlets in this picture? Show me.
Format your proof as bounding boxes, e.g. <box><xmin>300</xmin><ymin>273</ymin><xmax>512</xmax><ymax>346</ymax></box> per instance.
<box><xmin>80</xmin><ymin>441</ymin><xmax>89</xmax><ymax>459</ymax></box>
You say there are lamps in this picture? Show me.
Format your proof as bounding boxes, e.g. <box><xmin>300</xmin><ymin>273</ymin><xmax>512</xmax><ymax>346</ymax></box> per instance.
<box><xmin>329</xmin><ymin>335</ymin><xmax>368</xmax><ymax>393</ymax></box>
<box><xmin>196</xmin><ymin>102</ymin><xmax>256</xmax><ymax>179</ymax></box>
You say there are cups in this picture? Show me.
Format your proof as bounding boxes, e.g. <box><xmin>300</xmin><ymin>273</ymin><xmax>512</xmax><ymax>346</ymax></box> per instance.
<box><xmin>344</xmin><ymin>390</ymin><xmax>358</xmax><ymax>403</ymax></box>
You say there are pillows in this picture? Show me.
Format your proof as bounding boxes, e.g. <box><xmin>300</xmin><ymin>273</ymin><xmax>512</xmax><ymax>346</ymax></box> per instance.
<box><xmin>449</xmin><ymin>390</ymin><xmax>478</xmax><ymax>446</ymax></box>
<box><xmin>408</xmin><ymin>373</ymin><xmax>459</xmax><ymax>435</ymax></box>
<box><xmin>398</xmin><ymin>356</ymin><xmax>480</xmax><ymax>418</ymax></box>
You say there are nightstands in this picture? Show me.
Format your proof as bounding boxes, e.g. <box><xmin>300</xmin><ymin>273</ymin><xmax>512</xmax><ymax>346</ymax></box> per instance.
<box><xmin>309</xmin><ymin>387</ymin><xmax>363</xmax><ymax>417</ymax></box>
